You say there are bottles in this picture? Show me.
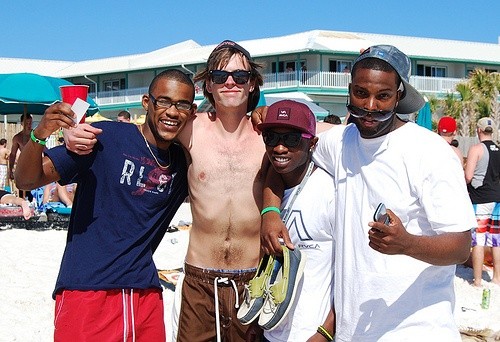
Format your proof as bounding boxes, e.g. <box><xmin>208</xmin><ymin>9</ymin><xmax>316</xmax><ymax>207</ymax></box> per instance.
<box><xmin>481</xmin><ymin>288</ymin><xmax>490</xmax><ymax>309</ymax></box>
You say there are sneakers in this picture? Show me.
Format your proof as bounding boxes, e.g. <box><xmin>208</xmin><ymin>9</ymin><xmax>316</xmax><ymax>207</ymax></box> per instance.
<box><xmin>237</xmin><ymin>254</ymin><xmax>274</xmax><ymax>325</ymax></box>
<box><xmin>258</xmin><ymin>245</ymin><xmax>305</xmax><ymax>331</ymax></box>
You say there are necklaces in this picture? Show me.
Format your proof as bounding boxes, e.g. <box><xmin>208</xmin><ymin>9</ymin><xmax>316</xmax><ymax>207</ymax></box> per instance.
<box><xmin>135</xmin><ymin>122</ymin><xmax>173</xmax><ymax>169</ymax></box>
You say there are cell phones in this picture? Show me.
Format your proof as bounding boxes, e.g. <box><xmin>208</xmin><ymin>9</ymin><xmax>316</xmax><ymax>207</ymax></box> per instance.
<box><xmin>373</xmin><ymin>203</ymin><xmax>390</xmax><ymax>225</ymax></box>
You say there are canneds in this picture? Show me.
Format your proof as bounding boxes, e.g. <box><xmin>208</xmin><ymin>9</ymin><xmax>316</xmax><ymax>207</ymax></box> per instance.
<box><xmin>482</xmin><ymin>288</ymin><xmax>490</xmax><ymax>309</ymax></box>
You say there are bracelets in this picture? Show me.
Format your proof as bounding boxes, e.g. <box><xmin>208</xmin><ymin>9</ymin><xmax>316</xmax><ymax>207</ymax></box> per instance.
<box><xmin>315</xmin><ymin>324</ymin><xmax>334</xmax><ymax>341</ymax></box>
<box><xmin>260</xmin><ymin>206</ymin><xmax>282</xmax><ymax>217</ymax></box>
<box><xmin>29</xmin><ymin>124</ymin><xmax>49</xmax><ymax>145</ymax></box>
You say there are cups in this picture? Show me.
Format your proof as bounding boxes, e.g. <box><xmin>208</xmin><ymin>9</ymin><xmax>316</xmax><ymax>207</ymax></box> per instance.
<box><xmin>59</xmin><ymin>84</ymin><xmax>89</xmax><ymax>123</ymax></box>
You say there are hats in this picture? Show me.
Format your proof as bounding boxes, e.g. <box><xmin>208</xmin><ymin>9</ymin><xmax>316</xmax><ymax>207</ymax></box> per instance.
<box><xmin>353</xmin><ymin>45</ymin><xmax>425</xmax><ymax>114</ymax></box>
<box><xmin>213</xmin><ymin>40</ymin><xmax>250</xmax><ymax>60</ymax></box>
<box><xmin>256</xmin><ymin>100</ymin><xmax>316</xmax><ymax>137</ymax></box>
<box><xmin>437</xmin><ymin>116</ymin><xmax>457</xmax><ymax>133</ymax></box>
<box><xmin>477</xmin><ymin>117</ymin><xmax>496</xmax><ymax>132</ymax></box>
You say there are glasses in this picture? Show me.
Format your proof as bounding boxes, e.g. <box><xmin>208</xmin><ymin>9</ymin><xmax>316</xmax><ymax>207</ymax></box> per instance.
<box><xmin>261</xmin><ymin>131</ymin><xmax>312</xmax><ymax>148</ymax></box>
<box><xmin>346</xmin><ymin>85</ymin><xmax>398</xmax><ymax>121</ymax></box>
<box><xmin>149</xmin><ymin>93</ymin><xmax>193</xmax><ymax>111</ymax></box>
<box><xmin>208</xmin><ymin>69</ymin><xmax>252</xmax><ymax>84</ymax></box>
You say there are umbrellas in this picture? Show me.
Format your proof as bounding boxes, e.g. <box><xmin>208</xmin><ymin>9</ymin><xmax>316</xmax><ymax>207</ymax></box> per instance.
<box><xmin>0</xmin><ymin>73</ymin><xmax>100</xmax><ymax>145</ymax></box>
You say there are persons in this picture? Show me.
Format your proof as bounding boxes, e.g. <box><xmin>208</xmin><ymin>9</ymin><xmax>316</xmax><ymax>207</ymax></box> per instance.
<box><xmin>14</xmin><ymin>41</ymin><xmax>267</xmax><ymax>342</ymax></box>
<box><xmin>342</xmin><ymin>64</ymin><xmax>351</xmax><ymax>87</ymax></box>
<box><xmin>0</xmin><ymin>114</ymin><xmax>78</xmax><ymax>221</ymax></box>
<box><xmin>284</xmin><ymin>61</ymin><xmax>307</xmax><ymax>85</ymax></box>
<box><xmin>323</xmin><ymin>111</ymin><xmax>351</xmax><ymax>126</ymax></box>
<box><xmin>61</xmin><ymin>41</ymin><xmax>271</xmax><ymax>342</ymax></box>
<box><xmin>260</xmin><ymin>45</ymin><xmax>479</xmax><ymax>342</ymax></box>
<box><xmin>436</xmin><ymin>116</ymin><xmax>500</xmax><ymax>289</ymax></box>
<box><xmin>116</xmin><ymin>109</ymin><xmax>131</xmax><ymax>123</ymax></box>
<box><xmin>257</xmin><ymin>99</ymin><xmax>336</xmax><ymax>342</ymax></box>
